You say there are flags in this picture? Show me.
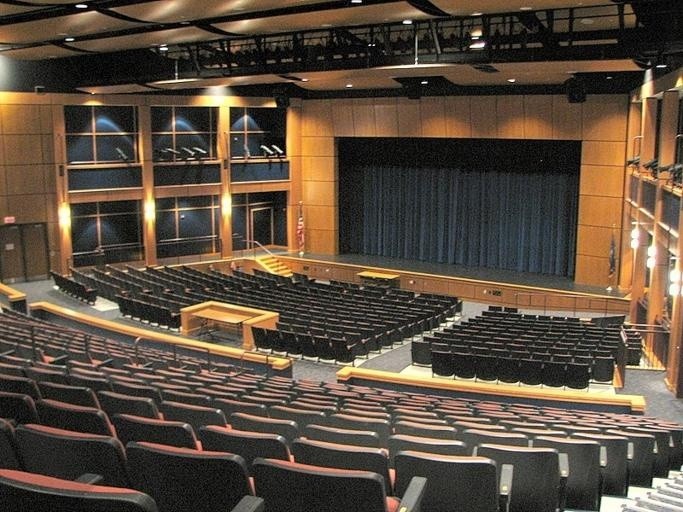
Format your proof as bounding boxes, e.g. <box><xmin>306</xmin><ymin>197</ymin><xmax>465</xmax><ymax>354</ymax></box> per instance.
<box><xmin>295</xmin><ymin>211</ymin><xmax>304</xmax><ymax>250</ymax></box>
<box><xmin>607</xmin><ymin>233</ymin><xmax>615</xmax><ymax>281</ymax></box>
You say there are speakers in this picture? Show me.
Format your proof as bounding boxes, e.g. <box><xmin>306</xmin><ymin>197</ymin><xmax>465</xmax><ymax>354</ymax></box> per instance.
<box><xmin>405</xmin><ymin>80</ymin><xmax>421</xmax><ymax>99</ymax></box>
<box><xmin>563</xmin><ymin>77</ymin><xmax>586</xmax><ymax>103</ymax></box>
<box><xmin>272</xmin><ymin>86</ymin><xmax>290</xmax><ymax>110</ymax></box>
